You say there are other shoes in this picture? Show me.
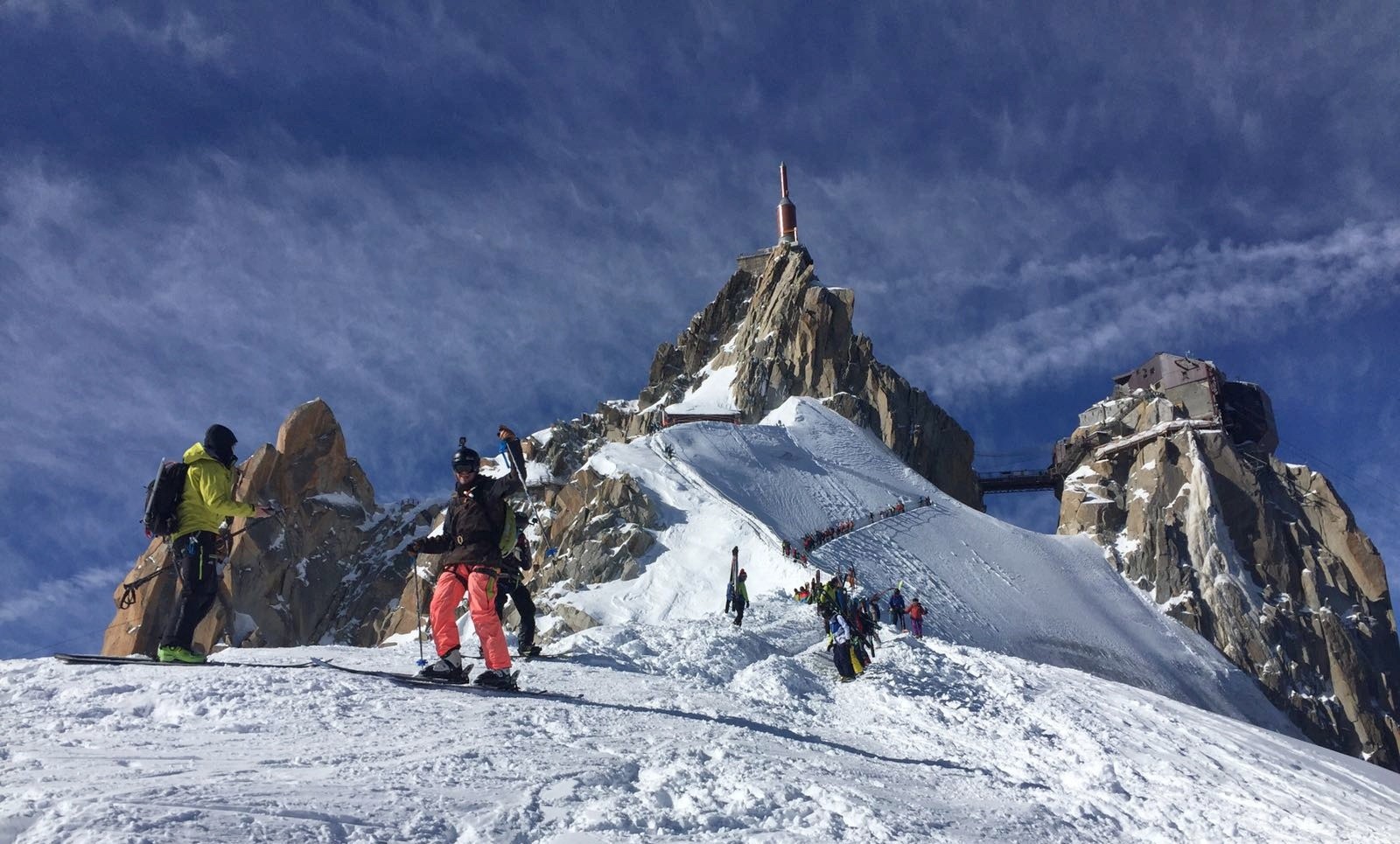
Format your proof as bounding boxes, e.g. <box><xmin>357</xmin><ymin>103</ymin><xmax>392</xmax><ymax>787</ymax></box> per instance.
<box><xmin>840</xmin><ymin>676</ymin><xmax>855</xmax><ymax>683</ymax></box>
<box><xmin>855</xmin><ymin>671</ymin><xmax>864</xmax><ymax>678</ymax></box>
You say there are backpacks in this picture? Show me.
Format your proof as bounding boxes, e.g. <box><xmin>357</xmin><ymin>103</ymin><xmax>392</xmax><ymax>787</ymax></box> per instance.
<box><xmin>142</xmin><ymin>461</ymin><xmax>188</xmax><ymax>537</ymax></box>
<box><xmin>498</xmin><ymin>503</ymin><xmax>519</xmax><ymax>558</ymax></box>
<box><xmin>727</xmin><ymin>582</ymin><xmax>735</xmax><ymax>602</ymax></box>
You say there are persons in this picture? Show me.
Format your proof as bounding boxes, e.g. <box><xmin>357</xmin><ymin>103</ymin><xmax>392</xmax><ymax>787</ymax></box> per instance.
<box><xmin>793</xmin><ymin>586</ymin><xmax>809</xmax><ymax>601</ymax></box>
<box><xmin>494</xmin><ymin>511</ymin><xmax>540</xmax><ymax>653</ymax></box>
<box><xmin>158</xmin><ymin>423</ymin><xmax>272</xmax><ymax>667</ymax></box>
<box><xmin>782</xmin><ymin>539</ymin><xmax>806</xmax><ymax>565</ymax></box>
<box><xmin>880</xmin><ymin>501</ymin><xmax>904</xmax><ymax>519</ymax></box>
<box><xmin>733</xmin><ymin>568</ymin><xmax>749</xmax><ymax>627</ymax></box>
<box><xmin>822</xmin><ymin>607</ymin><xmax>856</xmax><ymax>682</ymax></box>
<box><xmin>805</xmin><ymin>521</ymin><xmax>854</xmax><ymax>551</ymax></box>
<box><xmin>870</xmin><ymin>512</ymin><xmax>874</xmax><ymax>523</ymax></box>
<box><xmin>405</xmin><ymin>425</ymin><xmax>526</xmax><ymax>688</ymax></box>
<box><xmin>919</xmin><ymin>496</ymin><xmax>930</xmax><ymax>507</ymax></box>
<box><xmin>890</xmin><ymin>588</ymin><xmax>905</xmax><ymax>630</ymax></box>
<box><xmin>904</xmin><ymin>598</ymin><xmax>928</xmax><ymax>640</ymax></box>
<box><xmin>819</xmin><ymin>578</ymin><xmax>839</xmax><ymax>638</ymax></box>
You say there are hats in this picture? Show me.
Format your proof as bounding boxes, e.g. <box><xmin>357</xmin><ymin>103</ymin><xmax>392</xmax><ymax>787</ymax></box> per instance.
<box><xmin>739</xmin><ymin>569</ymin><xmax>746</xmax><ymax>577</ymax></box>
<box><xmin>205</xmin><ymin>424</ymin><xmax>237</xmax><ymax>449</ymax></box>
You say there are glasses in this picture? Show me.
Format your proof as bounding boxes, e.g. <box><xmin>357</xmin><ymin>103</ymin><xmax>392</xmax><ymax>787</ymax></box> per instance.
<box><xmin>454</xmin><ymin>463</ymin><xmax>473</xmax><ymax>472</ymax></box>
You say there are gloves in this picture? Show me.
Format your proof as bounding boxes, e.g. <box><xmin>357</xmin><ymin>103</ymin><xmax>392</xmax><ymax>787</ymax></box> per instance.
<box><xmin>845</xmin><ymin>640</ymin><xmax>851</xmax><ymax>649</ymax></box>
<box><xmin>828</xmin><ymin>643</ymin><xmax>833</xmax><ymax>652</ymax></box>
<box><xmin>407</xmin><ymin>538</ymin><xmax>424</xmax><ymax>556</ymax></box>
<box><xmin>499</xmin><ymin>427</ymin><xmax>515</xmax><ymax>441</ymax></box>
<box><xmin>732</xmin><ymin>602</ymin><xmax>736</xmax><ymax>611</ymax></box>
<box><xmin>746</xmin><ymin>601</ymin><xmax>749</xmax><ymax>608</ymax></box>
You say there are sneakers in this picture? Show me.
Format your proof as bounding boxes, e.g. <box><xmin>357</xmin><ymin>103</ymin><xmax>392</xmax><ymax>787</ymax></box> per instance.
<box><xmin>157</xmin><ymin>643</ymin><xmax>207</xmax><ymax>664</ymax></box>
<box><xmin>519</xmin><ymin>642</ymin><xmax>540</xmax><ymax>654</ymax></box>
<box><xmin>478</xmin><ymin>668</ymin><xmax>511</xmax><ymax>683</ymax></box>
<box><xmin>424</xmin><ymin>649</ymin><xmax>462</xmax><ymax>673</ymax></box>
<box><xmin>479</xmin><ymin>647</ymin><xmax>484</xmax><ymax>659</ymax></box>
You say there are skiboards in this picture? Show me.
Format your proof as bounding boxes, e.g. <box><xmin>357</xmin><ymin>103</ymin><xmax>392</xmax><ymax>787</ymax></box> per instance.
<box><xmin>725</xmin><ymin>546</ymin><xmax>738</xmax><ymax>613</ymax></box>
<box><xmin>470</xmin><ymin>646</ymin><xmax>542</xmax><ymax>658</ymax></box>
<box><xmin>807</xmin><ymin>561</ymin><xmax>903</xmax><ymax>657</ymax></box>
<box><xmin>310</xmin><ymin>656</ymin><xmax>584</xmax><ymax>699</ymax></box>
<box><xmin>54</xmin><ymin>653</ymin><xmax>333</xmax><ymax>668</ymax></box>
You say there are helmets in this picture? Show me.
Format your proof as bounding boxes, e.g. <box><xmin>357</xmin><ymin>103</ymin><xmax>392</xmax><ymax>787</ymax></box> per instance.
<box><xmin>452</xmin><ymin>447</ymin><xmax>480</xmax><ymax>473</ymax></box>
<box><xmin>516</xmin><ymin>512</ymin><xmax>529</xmax><ymax>531</ymax></box>
